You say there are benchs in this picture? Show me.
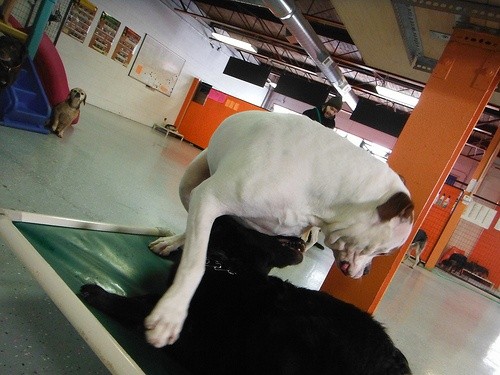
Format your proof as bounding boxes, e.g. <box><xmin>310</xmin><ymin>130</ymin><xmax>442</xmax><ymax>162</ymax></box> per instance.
<box><xmin>463</xmin><ymin>268</ymin><xmax>496</xmax><ymax>289</ymax></box>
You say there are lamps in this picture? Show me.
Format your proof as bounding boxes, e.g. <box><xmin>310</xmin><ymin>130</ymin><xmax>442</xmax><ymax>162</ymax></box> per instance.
<box><xmin>377</xmin><ymin>80</ymin><xmax>424</xmax><ymax>107</ymax></box>
<box><xmin>211</xmin><ymin>32</ymin><xmax>258</xmax><ymax>53</ymax></box>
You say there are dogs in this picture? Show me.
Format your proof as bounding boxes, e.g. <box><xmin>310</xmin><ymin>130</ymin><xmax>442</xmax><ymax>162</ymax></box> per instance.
<box><xmin>400</xmin><ymin>229</ymin><xmax>428</xmax><ymax>269</ymax></box>
<box><xmin>80</xmin><ymin>217</ymin><xmax>411</xmax><ymax>374</ymax></box>
<box><xmin>0</xmin><ymin>36</ymin><xmax>30</xmax><ymax>90</ymax></box>
<box><xmin>51</xmin><ymin>87</ymin><xmax>87</xmax><ymax>138</ymax></box>
<box><xmin>144</xmin><ymin>109</ymin><xmax>416</xmax><ymax>349</ymax></box>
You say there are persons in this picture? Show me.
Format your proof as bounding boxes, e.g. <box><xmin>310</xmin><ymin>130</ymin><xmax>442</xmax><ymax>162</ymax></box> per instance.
<box><xmin>302</xmin><ymin>96</ymin><xmax>342</xmax><ymax>129</ymax></box>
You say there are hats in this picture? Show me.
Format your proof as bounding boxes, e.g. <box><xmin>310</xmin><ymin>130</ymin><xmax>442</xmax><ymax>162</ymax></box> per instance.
<box><xmin>326</xmin><ymin>96</ymin><xmax>342</xmax><ymax>112</ymax></box>
